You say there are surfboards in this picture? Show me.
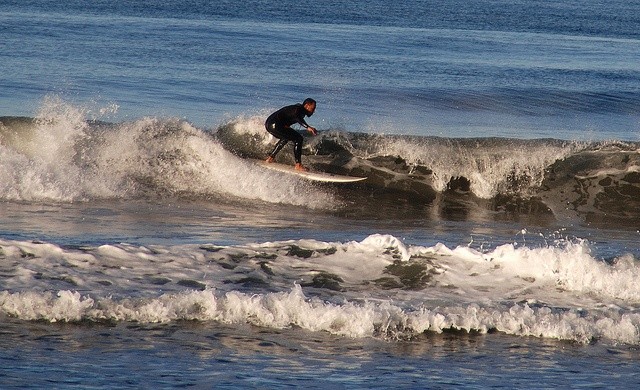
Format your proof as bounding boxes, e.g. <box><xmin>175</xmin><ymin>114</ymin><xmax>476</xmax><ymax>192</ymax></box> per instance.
<box><xmin>245</xmin><ymin>159</ymin><xmax>368</xmax><ymax>183</ymax></box>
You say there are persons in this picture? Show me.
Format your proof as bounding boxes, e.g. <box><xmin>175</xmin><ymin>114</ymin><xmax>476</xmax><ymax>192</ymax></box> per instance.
<box><xmin>265</xmin><ymin>98</ymin><xmax>318</xmax><ymax>171</ymax></box>
